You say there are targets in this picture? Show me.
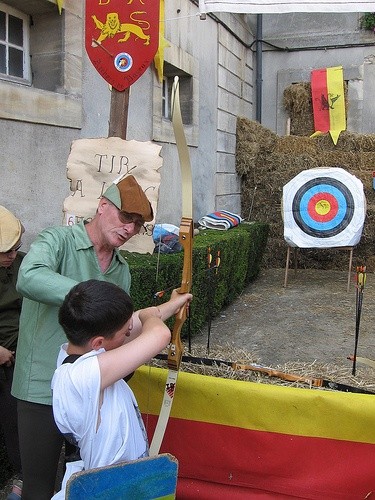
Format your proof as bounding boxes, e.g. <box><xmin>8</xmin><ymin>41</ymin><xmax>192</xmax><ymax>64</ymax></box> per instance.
<box><xmin>281</xmin><ymin>167</ymin><xmax>367</xmax><ymax>247</ymax></box>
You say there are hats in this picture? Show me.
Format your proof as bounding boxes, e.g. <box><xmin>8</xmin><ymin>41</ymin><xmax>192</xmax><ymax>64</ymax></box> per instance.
<box><xmin>0</xmin><ymin>205</ymin><xmax>25</xmax><ymax>253</ymax></box>
<box><xmin>102</xmin><ymin>175</ymin><xmax>154</xmax><ymax>222</ymax></box>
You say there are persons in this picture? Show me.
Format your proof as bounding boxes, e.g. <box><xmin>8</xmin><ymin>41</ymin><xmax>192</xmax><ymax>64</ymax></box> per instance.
<box><xmin>0</xmin><ymin>204</ymin><xmax>27</xmax><ymax>489</ymax></box>
<box><xmin>11</xmin><ymin>175</ymin><xmax>154</xmax><ymax>500</ymax></box>
<box><xmin>49</xmin><ymin>279</ymin><xmax>193</xmax><ymax>500</ymax></box>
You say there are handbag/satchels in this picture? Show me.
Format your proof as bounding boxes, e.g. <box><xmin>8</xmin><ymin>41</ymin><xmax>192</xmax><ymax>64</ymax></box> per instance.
<box><xmin>153</xmin><ymin>224</ymin><xmax>183</xmax><ymax>254</ymax></box>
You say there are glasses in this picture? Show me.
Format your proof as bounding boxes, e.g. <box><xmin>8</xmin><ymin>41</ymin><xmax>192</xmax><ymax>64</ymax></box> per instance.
<box><xmin>119</xmin><ymin>212</ymin><xmax>148</xmax><ymax>233</ymax></box>
<box><xmin>7</xmin><ymin>242</ymin><xmax>23</xmax><ymax>254</ymax></box>
